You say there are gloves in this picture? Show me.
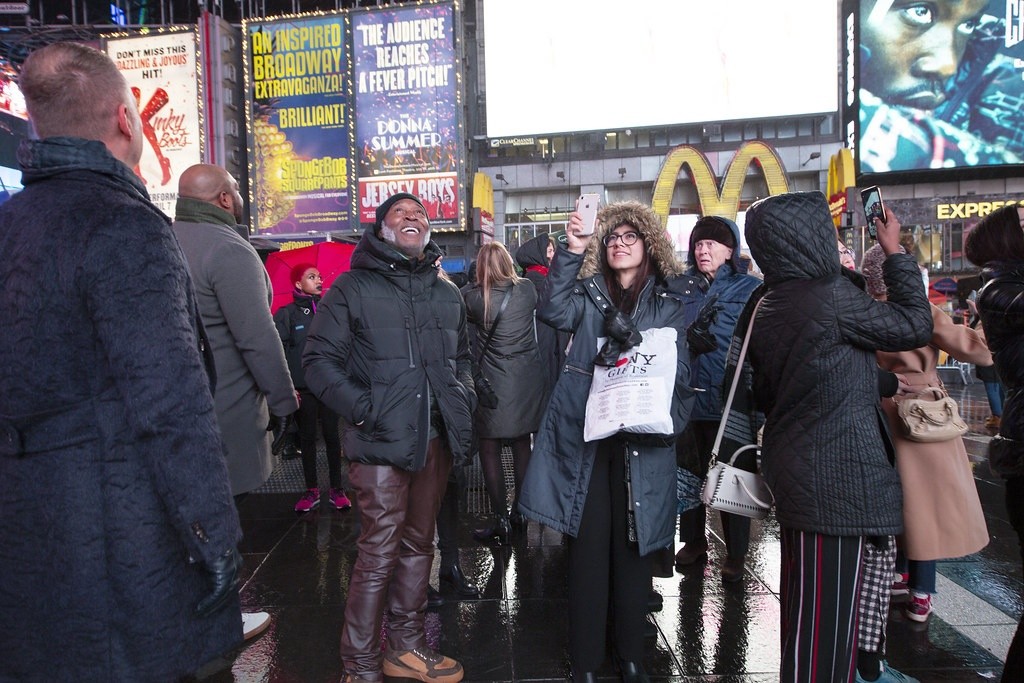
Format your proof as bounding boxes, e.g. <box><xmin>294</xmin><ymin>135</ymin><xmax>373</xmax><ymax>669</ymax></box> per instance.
<box><xmin>692</xmin><ymin>297</ymin><xmax>725</xmax><ymax>335</ymax></box>
<box><xmin>592</xmin><ymin>293</ymin><xmax>644</xmax><ymax>368</ymax></box>
<box><xmin>196</xmin><ymin>546</ymin><xmax>244</xmax><ymax>618</ymax></box>
<box><xmin>266</xmin><ymin>414</ymin><xmax>290</xmax><ymax>456</ymax></box>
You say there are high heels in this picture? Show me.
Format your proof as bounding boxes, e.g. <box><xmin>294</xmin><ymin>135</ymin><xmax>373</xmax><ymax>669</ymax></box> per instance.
<box><xmin>570</xmin><ymin>661</ymin><xmax>598</xmax><ymax>683</ymax></box>
<box><xmin>612</xmin><ymin>648</ymin><xmax>650</xmax><ymax>683</ymax></box>
<box><xmin>474</xmin><ymin>514</ymin><xmax>511</xmax><ymax>545</ymax></box>
<box><xmin>510</xmin><ymin>510</ymin><xmax>527</xmax><ymax>533</ymax></box>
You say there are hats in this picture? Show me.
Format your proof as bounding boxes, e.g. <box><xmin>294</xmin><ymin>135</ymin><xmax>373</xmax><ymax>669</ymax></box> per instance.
<box><xmin>290</xmin><ymin>262</ymin><xmax>318</xmax><ymax>288</ymax></box>
<box><xmin>373</xmin><ymin>193</ymin><xmax>429</xmax><ymax>236</ymax></box>
<box><xmin>691</xmin><ymin>216</ymin><xmax>736</xmax><ymax>251</ymax></box>
<box><xmin>859</xmin><ymin>243</ymin><xmax>907</xmax><ymax>295</ymax></box>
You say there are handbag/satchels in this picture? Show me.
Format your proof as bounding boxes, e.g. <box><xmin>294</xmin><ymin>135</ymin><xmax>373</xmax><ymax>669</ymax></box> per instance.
<box><xmin>896</xmin><ymin>387</ymin><xmax>970</xmax><ymax>443</ymax></box>
<box><xmin>475</xmin><ymin>376</ymin><xmax>499</xmax><ymax>409</ymax></box>
<box><xmin>583</xmin><ymin>325</ymin><xmax>678</xmax><ymax>443</ymax></box>
<box><xmin>699</xmin><ymin>445</ymin><xmax>777</xmax><ymax>520</ymax></box>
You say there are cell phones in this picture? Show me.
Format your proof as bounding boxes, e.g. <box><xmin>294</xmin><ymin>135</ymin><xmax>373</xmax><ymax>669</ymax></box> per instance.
<box><xmin>967</xmin><ymin>290</ymin><xmax>976</xmax><ymax>314</ymax></box>
<box><xmin>574</xmin><ymin>193</ymin><xmax>600</xmax><ymax>236</ymax></box>
<box><xmin>860</xmin><ymin>185</ymin><xmax>887</xmax><ymax>240</ymax></box>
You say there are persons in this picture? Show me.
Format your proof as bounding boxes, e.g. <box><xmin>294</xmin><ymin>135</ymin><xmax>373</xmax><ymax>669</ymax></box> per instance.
<box><xmin>467</xmin><ymin>241</ymin><xmax>540</xmax><ymax>543</ymax></box>
<box><xmin>861</xmin><ymin>0</ymin><xmax>1022</xmax><ymax>172</ymax></box>
<box><xmin>415</xmin><ymin>240</ymin><xmax>475</xmax><ymax>608</ymax></box>
<box><xmin>860</xmin><ymin>241</ymin><xmax>995</xmax><ymax>622</ymax></box>
<box><xmin>969</xmin><ymin>269</ymin><xmax>1003</xmax><ymax>428</ymax></box>
<box><xmin>2</xmin><ymin>42</ymin><xmax>245</xmax><ymax>681</ymax></box>
<box><xmin>517</xmin><ymin>191</ymin><xmax>690</xmax><ymax>682</ymax></box>
<box><xmin>447</xmin><ymin>261</ymin><xmax>475</xmax><ymax>292</ymax></box>
<box><xmin>300</xmin><ymin>193</ymin><xmax>476</xmax><ymax>682</ymax></box>
<box><xmin>674</xmin><ymin>214</ymin><xmax>765</xmax><ymax>584</ymax></box>
<box><xmin>515</xmin><ymin>234</ymin><xmax>562</xmax><ymax>386</ymax></box>
<box><xmin>697</xmin><ymin>186</ymin><xmax>932</xmax><ymax>683</ymax></box>
<box><xmin>829</xmin><ymin>235</ymin><xmax>920</xmax><ymax>683</ymax></box>
<box><xmin>168</xmin><ymin>165</ymin><xmax>298</xmax><ymax>639</ymax></box>
<box><xmin>270</xmin><ymin>262</ymin><xmax>351</xmax><ymax>514</ymax></box>
<box><xmin>964</xmin><ymin>204</ymin><xmax>1023</xmax><ymax>683</ymax></box>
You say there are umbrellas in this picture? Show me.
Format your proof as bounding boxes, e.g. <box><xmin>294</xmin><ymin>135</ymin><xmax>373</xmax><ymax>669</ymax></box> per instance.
<box><xmin>260</xmin><ymin>243</ymin><xmax>355</xmax><ymax>316</ymax></box>
<box><xmin>927</xmin><ymin>276</ymin><xmax>958</xmax><ymax>305</ymax></box>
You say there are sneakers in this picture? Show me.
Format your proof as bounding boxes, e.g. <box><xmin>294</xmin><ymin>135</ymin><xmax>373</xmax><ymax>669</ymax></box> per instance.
<box><xmin>891</xmin><ymin>574</ymin><xmax>910</xmax><ymax>595</ymax></box>
<box><xmin>328</xmin><ymin>486</ymin><xmax>352</xmax><ymax>510</ymax></box>
<box><xmin>854</xmin><ymin>660</ymin><xmax>921</xmax><ymax>683</ymax></box>
<box><xmin>675</xmin><ymin>535</ymin><xmax>708</xmax><ymax>566</ymax></box>
<box><xmin>294</xmin><ymin>487</ymin><xmax>321</xmax><ymax>512</ymax></box>
<box><xmin>905</xmin><ymin>592</ymin><xmax>935</xmax><ymax>622</ymax></box>
<box><xmin>339</xmin><ymin>672</ymin><xmax>384</xmax><ymax>682</ymax></box>
<box><xmin>383</xmin><ymin>644</ymin><xmax>463</xmax><ymax>683</ymax></box>
<box><xmin>722</xmin><ymin>553</ymin><xmax>746</xmax><ymax>581</ymax></box>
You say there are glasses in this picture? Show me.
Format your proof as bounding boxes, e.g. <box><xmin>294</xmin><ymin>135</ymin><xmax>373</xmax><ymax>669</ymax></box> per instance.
<box><xmin>602</xmin><ymin>231</ymin><xmax>645</xmax><ymax>248</ymax></box>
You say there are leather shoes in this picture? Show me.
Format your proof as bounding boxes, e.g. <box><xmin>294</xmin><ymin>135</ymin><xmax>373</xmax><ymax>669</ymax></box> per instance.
<box><xmin>239</xmin><ymin>611</ymin><xmax>271</xmax><ymax>641</ymax></box>
<box><xmin>439</xmin><ymin>563</ymin><xmax>478</xmax><ymax>595</ymax></box>
<box><xmin>427</xmin><ymin>582</ymin><xmax>446</xmax><ymax>606</ymax></box>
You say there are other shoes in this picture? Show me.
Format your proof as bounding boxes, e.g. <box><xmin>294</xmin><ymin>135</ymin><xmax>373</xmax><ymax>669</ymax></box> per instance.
<box><xmin>644</xmin><ymin>620</ymin><xmax>657</xmax><ymax>636</ymax></box>
<box><xmin>647</xmin><ymin>590</ymin><xmax>664</xmax><ymax>606</ymax></box>
